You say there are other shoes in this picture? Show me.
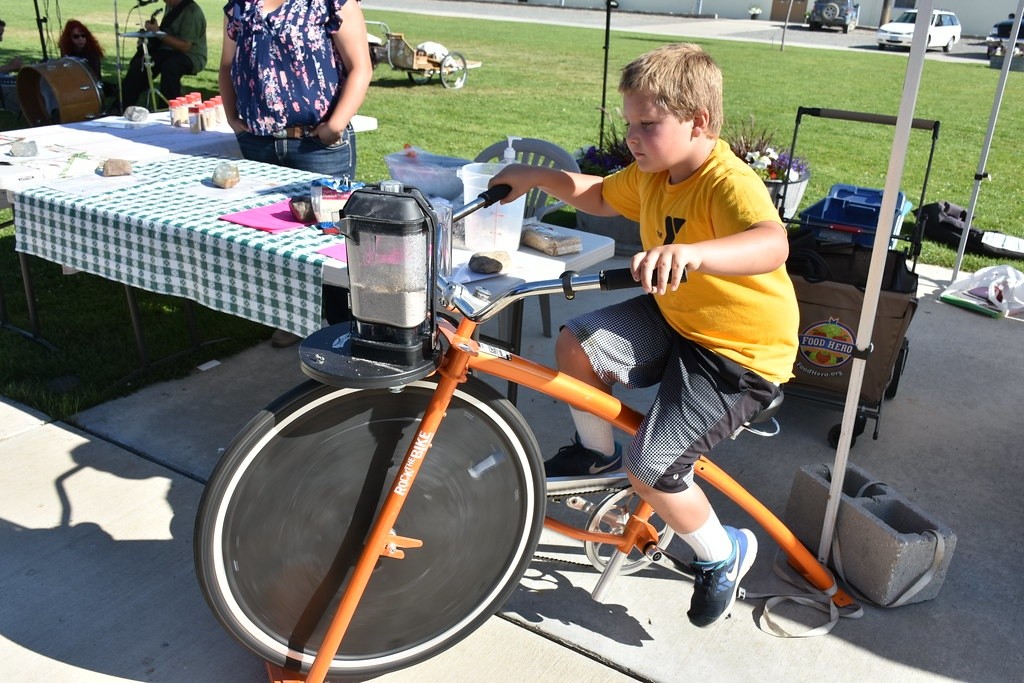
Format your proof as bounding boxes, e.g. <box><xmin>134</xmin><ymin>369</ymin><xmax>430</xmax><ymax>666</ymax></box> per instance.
<box><xmin>272</xmin><ymin>327</ymin><xmax>303</xmax><ymax>348</ymax></box>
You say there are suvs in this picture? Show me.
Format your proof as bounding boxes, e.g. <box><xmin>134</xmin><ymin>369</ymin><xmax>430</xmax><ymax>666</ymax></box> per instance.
<box><xmin>809</xmin><ymin>0</ymin><xmax>860</xmax><ymax>35</ymax></box>
<box><xmin>875</xmin><ymin>8</ymin><xmax>962</xmax><ymax>52</ymax></box>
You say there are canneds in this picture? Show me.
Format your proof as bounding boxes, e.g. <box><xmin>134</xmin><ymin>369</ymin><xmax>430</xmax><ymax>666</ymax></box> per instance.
<box><xmin>168</xmin><ymin>92</ymin><xmax>224</xmax><ymax>134</ymax></box>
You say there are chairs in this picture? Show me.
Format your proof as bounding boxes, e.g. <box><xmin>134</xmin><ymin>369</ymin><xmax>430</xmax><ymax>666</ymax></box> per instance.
<box><xmin>474</xmin><ymin>138</ymin><xmax>582</xmax><ymax>338</ymax></box>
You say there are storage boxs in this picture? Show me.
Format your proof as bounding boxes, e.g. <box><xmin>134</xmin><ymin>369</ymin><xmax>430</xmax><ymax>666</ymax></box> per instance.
<box><xmin>798</xmin><ymin>184</ymin><xmax>902</xmax><ymax>248</ymax></box>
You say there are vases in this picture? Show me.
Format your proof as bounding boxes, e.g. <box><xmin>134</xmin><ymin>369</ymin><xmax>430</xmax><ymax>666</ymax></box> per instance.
<box><xmin>763</xmin><ymin>177</ymin><xmax>809</xmax><ymax>231</ymax></box>
<box><xmin>990</xmin><ymin>56</ymin><xmax>1024</xmax><ymax>71</ymax></box>
<box><xmin>572</xmin><ymin>147</ymin><xmax>645</xmax><ymax>258</ymax></box>
<box><xmin>751</xmin><ymin>14</ymin><xmax>759</xmax><ymax>19</ymax></box>
<box><xmin>804</xmin><ymin>18</ymin><xmax>810</xmax><ymax>24</ymax></box>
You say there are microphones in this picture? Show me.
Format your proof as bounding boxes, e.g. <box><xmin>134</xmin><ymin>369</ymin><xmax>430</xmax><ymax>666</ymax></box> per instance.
<box><xmin>134</xmin><ymin>0</ymin><xmax>158</xmax><ymax>8</ymax></box>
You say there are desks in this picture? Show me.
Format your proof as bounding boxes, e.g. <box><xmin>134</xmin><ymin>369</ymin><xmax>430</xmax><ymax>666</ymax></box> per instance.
<box><xmin>7</xmin><ymin>150</ymin><xmax>614</xmax><ymax>416</ymax></box>
<box><xmin>0</xmin><ymin>112</ymin><xmax>377</xmax><ymax>357</ymax></box>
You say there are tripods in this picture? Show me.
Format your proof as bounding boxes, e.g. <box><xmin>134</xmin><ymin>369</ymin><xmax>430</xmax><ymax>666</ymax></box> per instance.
<box><xmin>140</xmin><ymin>37</ymin><xmax>171</xmax><ymax>113</ymax></box>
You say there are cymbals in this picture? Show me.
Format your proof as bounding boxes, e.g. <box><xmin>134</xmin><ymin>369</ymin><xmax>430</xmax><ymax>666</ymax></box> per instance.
<box><xmin>119</xmin><ymin>31</ymin><xmax>167</xmax><ymax>38</ymax></box>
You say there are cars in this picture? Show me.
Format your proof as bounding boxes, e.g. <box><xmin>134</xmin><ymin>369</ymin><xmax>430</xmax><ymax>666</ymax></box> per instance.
<box><xmin>986</xmin><ymin>11</ymin><xmax>1024</xmax><ymax>59</ymax></box>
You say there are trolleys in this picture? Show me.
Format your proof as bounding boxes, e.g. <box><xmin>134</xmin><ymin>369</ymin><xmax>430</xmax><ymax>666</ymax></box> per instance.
<box><xmin>775</xmin><ymin>105</ymin><xmax>941</xmax><ymax>460</ymax></box>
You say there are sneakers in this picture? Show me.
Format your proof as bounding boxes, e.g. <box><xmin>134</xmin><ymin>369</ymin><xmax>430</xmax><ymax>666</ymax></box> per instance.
<box><xmin>543</xmin><ymin>431</ymin><xmax>623</xmax><ymax>477</ymax></box>
<box><xmin>687</xmin><ymin>525</ymin><xmax>759</xmax><ymax>629</ymax></box>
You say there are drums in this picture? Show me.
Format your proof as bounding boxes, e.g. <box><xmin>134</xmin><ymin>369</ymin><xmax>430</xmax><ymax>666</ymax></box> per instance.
<box><xmin>15</xmin><ymin>55</ymin><xmax>107</xmax><ymax>128</ymax></box>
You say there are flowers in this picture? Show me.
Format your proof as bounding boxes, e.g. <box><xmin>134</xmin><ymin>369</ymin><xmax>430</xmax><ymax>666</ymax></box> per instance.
<box><xmin>723</xmin><ymin>114</ymin><xmax>811</xmax><ymax>181</ymax></box>
<box><xmin>804</xmin><ymin>11</ymin><xmax>812</xmax><ymax>19</ymax></box>
<box><xmin>572</xmin><ymin>106</ymin><xmax>635</xmax><ymax>177</ymax></box>
<box><xmin>988</xmin><ymin>38</ymin><xmax>1024</xmax><ymax>57</ymax></box>
<box><xmin>748</xmin><ymin>4</ymin><xmax>762</xmax><ymax>15</ymax></box>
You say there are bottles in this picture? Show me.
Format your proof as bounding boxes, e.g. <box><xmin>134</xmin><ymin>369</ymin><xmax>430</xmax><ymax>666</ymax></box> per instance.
<box><xmin>169</xmin><ymin>92</ymin><xmax>227</xmax><ymax>133</ymax></box>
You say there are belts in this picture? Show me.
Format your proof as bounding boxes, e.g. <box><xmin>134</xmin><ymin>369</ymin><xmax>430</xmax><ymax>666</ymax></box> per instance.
<box><xmin>272</xmin><ymin>125</ymin><xmax>307</xmax><ymax>140</ymax></box>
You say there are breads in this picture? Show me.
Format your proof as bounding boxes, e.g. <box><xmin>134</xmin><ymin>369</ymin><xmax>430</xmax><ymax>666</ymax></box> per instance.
<box><xmin>519</xmin><ymin>223</ymin><xmax>583</xmax><ymax>256</ymax></box>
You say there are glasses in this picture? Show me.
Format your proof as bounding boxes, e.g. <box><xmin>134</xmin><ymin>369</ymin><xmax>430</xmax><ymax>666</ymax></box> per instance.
<box><xmin>70</xmin><ymin>33</ymin><xmax>87</xmax><ymax>39</ymax></box>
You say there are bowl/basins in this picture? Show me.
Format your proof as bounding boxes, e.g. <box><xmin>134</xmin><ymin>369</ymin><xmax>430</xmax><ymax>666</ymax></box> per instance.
<box><xmin>386</xmin><ymin>152</ymin><xmax>471</xmax><ymax>202</ymax></box>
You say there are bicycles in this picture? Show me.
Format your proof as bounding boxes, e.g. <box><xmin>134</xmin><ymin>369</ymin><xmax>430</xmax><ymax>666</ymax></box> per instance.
<box><xmin>191</xmin><ymin>171</ymin><xmax>854</xmax><ymax>683</ymax></box>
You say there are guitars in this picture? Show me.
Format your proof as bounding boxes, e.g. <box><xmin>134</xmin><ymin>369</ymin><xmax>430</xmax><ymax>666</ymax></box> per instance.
<box><xmin>129</xmin><ymin>7</ymin><xmax>164</xmax><ymax>69</ymax></box>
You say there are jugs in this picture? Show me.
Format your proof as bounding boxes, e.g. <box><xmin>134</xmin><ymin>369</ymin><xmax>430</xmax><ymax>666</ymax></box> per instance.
<box><xmin>456</xmin><ymin>162</ymin><xmax>526</xmax><ymax>253</ymax></box>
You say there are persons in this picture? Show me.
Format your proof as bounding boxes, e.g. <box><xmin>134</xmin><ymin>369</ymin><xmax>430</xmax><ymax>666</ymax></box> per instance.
<box><xmin>58</xmin><ymin>19</ymin><xmax>104</xmax><ymax>78</ymax></box>
<box><xmin>219</xmin><ymin>0</ymin><xmax>372</xmax><ymax>347</ymax></box>
<box><xmin>108</xmin><ymin>0</ymin><xmax>208</xmax><ymax>116</ymax></box>
<box><xmin>0</xmin><ymin>19</ymin><xmax>25</xmax><ymax>78</ymax></box>
<box><xmin>486</xmin><ymin>41</ymin><xmax>802</xmax><ymax>628</ymax></box>
